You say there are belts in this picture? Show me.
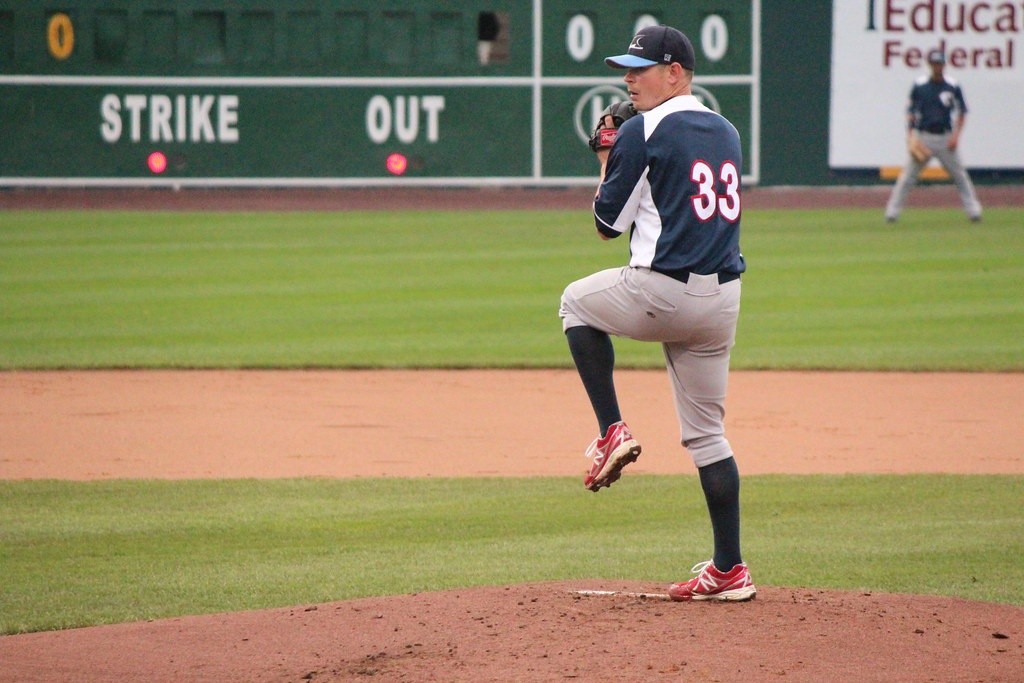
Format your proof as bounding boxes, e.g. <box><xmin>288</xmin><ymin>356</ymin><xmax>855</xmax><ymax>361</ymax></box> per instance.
<box><xmin>914</xmin><ymin>123</ymin><xmax>951</xmax><ymax>134</ymax></box>
<box><xmin>650</xmin><ymin>266</ymin><xmax>741</xmax><ymax>285</ymax></box>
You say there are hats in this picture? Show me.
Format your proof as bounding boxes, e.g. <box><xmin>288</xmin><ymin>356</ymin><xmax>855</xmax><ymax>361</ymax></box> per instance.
<box><xmin>928</xmin><ymin>52</ymin><xmax>945</xmax><ymax>64</ymax></box>
<box><xmin>604</xmin><ymin>24</ymin><xmax>695</xmax><ymax>71</ymax></box>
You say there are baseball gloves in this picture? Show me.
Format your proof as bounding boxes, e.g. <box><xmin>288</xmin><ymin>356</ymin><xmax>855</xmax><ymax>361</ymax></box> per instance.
<box><xmin>589</xmin><ymin>100</ymin><xmax>640</xmax><ymax>153</ymax></box>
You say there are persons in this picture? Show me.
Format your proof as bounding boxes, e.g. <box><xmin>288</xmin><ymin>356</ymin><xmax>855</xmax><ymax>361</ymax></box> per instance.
<box><xmin>559</xmin><ymin>25</ymin><xmax>758</xmax><ymax>604</ymax></box>
<box><xmin>885</xmin><ymin>50</ymin><xmax>983</xmax><ymax>223</ymax></box>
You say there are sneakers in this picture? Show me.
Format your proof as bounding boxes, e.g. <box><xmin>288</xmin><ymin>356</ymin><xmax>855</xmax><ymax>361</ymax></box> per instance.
<box><xmin>583</xmin><ymin>421</ymin><xmax>641</xmax><ymax>492</ymax></box>
<box><xmin>669</xmin><ymin>559</ymin><xmax>756</xmax><ymax>601</ymax></box>
